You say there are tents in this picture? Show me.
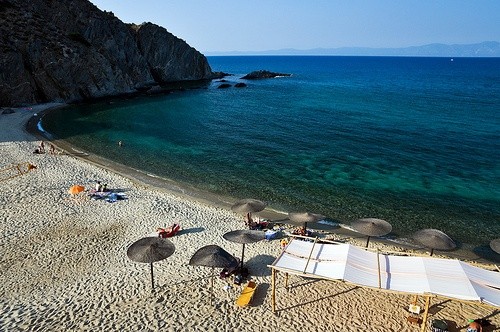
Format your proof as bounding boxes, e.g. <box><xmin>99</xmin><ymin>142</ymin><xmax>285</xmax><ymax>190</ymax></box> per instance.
<box><xmin>267</xmin><ymin>239</ymin><xmax>500</xmax><ymax>332</ymax></box>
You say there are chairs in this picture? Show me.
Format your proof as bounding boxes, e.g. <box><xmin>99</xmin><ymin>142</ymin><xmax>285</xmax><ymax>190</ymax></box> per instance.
<box><xmin>428</xmin><ymin>319</ymin><xmax>448</xmax><ymax>332</ymax></box>
<box><xmin>235</xmin><ymin>281</ymin><xmax>258</xmax><ymax>309</ymax></box>
<box><xmin>157</xmin><ymin>223</ymin><xmax>180</xmax><ymax>238</ymax></box>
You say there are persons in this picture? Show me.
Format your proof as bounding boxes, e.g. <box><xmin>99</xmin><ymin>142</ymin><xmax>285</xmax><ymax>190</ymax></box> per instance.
<box><xmin>49</xmin><ymin>144</ymin><xmax>55</xmax><ymax>152</ymax></box>
<box><xmin>96</xmin><ymin>181</ymin><xmax>109</xmax><ymax>192</ymax></box>
<box><xmin>247</xmin><ymin>214</ymin><xmax>306</xmax><ymax>235</ymax></box>
<box><xmin>157</xmin><ymin>224</ymin><xmax>177</xmax><ymax>237</ymax></box>
<box><xmin>40</xmin><ymin>140</ymin><xmax>44</xmax><ymax>149</ymax></box>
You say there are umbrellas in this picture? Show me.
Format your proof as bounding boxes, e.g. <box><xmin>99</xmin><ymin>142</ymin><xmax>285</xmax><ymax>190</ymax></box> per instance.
<box><xmin>351</xmin><ymin>217</ymin><xmax>392</xmax><ymax>247</ymax></box>
<box><xmin>71</xmin><ymin>185</ymin><xmax>84</xmax><ymax>194</ymax></box>
<box><xmin>127</xmin><ymin>237</ymin><xmax>176</xmax><ymax>286</ymax></box>
<box><xmin>489</xmin><ymin>238</ymin><xmax>500</xmax><ymax>254</ymax></box>
<box><xmin>232</xmin><ymin>199</ymin><xmax>266</xmax><ymax>218</ymax></box>
<box><xmin>222</xmin><ymin>229</ymin><xmax>264</xmax><ymax>266</ymax></box>
<box><xmin>415</xmin><ymin>228</ymin><xmax>456</xmax><ymax>256</ymax></box>
<box><xmin>287</xmin><ymin>211</ymin><xmax>326</xmax><ymax>231</ymax></box>
<box><xmin>189</xmin><ymin>245</ymin><xmax>238</xmax><ymax>286</ymax></box>
